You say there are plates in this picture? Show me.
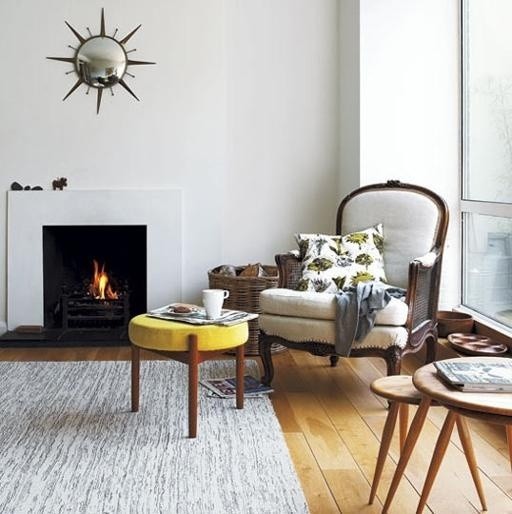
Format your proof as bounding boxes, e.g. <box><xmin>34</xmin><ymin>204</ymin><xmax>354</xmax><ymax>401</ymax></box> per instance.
<box><xmin>446</xmin><ymin>332</ymin><xmax>508</xmax><ymax>356</ymax></box>
<box><xmin>167</xmin><ymin>307</ymin><xmax>199</xmax><ymax>317</ymax></box>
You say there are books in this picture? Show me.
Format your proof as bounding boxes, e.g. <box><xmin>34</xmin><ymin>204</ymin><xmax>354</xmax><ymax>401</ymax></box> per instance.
<box><xmin>433</xmin><ymin>357</ymin><xmax>512</xmax><ymax>394</ymax></box>
<box><xmin>198</xmin><ymin>373</ymin><xmax>275</xmax><ymax>398</ymax></box>
<box><xmin>210</xmin><ymin>311</ymin><xmax>260</xmax><ymax>328</ymax></box>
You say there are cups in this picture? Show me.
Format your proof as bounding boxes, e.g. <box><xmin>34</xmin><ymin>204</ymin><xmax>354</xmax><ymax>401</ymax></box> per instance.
<box><xmin>202</xmin><ymin>289</ymin><xmax>230</xmax><ymax>320</ymax></box>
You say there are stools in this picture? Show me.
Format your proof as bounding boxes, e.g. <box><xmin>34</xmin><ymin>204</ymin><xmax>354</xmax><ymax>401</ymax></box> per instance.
<box><xmin>369</xmin><ymin>375</ymin><xmax>488</xmax><ymax>511</ymax></box>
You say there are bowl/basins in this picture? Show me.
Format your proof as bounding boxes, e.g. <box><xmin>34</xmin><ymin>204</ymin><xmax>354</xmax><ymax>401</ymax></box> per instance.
<box><xmin>437</xmin><ymin>311</ymin><xmax>474</xmax><ymax>338</ymax></box>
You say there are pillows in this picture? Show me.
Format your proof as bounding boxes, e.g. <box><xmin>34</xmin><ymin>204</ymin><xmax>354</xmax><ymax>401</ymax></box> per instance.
<box><xmin>294</xmin><ymin>223</ymin><xmax>386</xmax><ymax>294</ymax></box>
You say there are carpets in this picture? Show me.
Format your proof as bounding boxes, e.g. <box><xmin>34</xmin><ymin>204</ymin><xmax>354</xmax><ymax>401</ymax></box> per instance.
<box><xmin>0</xmin><ymin>360</ymin><xmax>310</xmax><ymax>514</ymax></box>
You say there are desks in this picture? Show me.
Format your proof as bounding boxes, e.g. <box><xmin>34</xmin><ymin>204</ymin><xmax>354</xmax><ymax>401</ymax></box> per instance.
<box><xmin>128</xmin><ymin>313</ymin><xmax>249</xmax><ymax>438</ymax></box>
<box><xmin>381</xmin><ymin>357</ymin><xmax>512</xmax><ymax>514</ymax></box>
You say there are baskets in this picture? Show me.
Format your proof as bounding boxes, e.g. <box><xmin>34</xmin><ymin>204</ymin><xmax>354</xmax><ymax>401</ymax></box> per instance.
<box><xmin>206</xmin><ymin>260</ymin><xmax>289</xmax><ymax>357</ymax></box>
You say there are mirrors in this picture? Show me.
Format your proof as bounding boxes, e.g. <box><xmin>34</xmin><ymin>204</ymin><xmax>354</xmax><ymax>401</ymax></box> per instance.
<box><xmin>44</xmin><ymin>7</ymin><xmax>156</xmax><ymax>115</ymax></box>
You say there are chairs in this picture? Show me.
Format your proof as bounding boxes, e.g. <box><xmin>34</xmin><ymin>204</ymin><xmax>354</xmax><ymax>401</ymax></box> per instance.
<box><xmin>256</xmin><ymin>179</ymin><xmax>449</xmax><ymax>410</ymax></box>
